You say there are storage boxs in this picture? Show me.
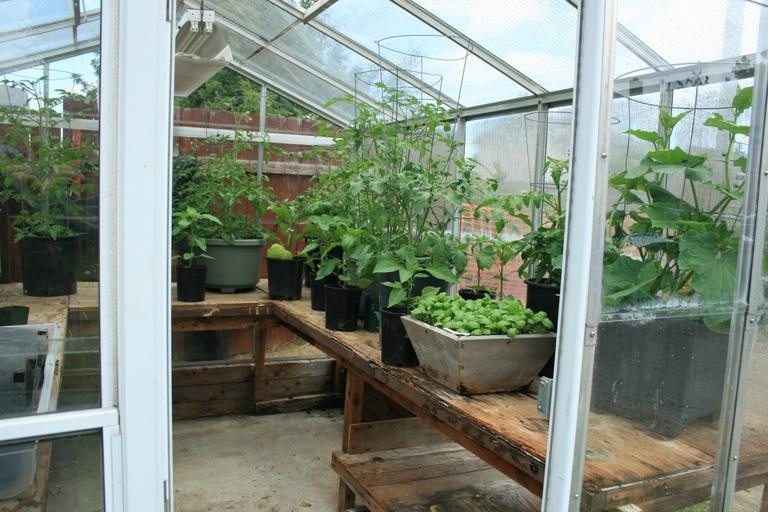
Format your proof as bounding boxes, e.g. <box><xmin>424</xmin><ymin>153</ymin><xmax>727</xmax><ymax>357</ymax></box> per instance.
<box><xmin>1</xmin><ymin>321</ymin><xmax>61</xmax><ymax>500</ymax></box>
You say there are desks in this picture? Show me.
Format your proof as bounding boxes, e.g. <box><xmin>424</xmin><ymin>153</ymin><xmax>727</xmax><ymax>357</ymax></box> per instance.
<box><xmin>273</xmin><ymin>300</ymin><xmax>764</xmax><ymax>512</ymax></box>
